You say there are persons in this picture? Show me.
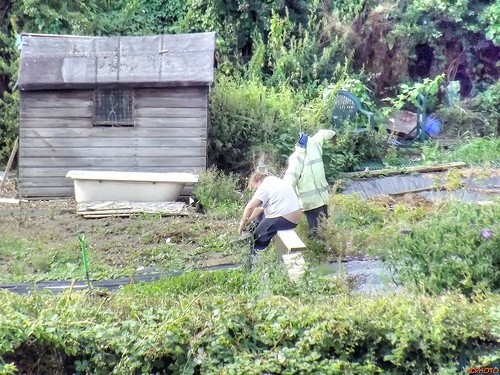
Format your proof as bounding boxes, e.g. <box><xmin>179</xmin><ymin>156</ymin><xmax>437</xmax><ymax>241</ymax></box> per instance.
<box><xmin>238</xmin><ymin>171</ymin><xmax>301</xmax><ymax>256</ymax></box>
<box><xmin>283</xmin><ymin>129</ymin><xmax>336</xmax><ymax>236</ymax></box>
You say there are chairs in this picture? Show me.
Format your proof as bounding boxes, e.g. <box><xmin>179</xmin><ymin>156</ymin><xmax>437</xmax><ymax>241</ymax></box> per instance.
<box><xmin>332</xmin><ymin>90</ymin><xmax>374</xmax><ymax>135</ymax></box>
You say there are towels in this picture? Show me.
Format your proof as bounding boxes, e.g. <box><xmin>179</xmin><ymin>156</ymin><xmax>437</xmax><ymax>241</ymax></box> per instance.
<box><xmin>66</xmin><ymin>169</ymin><xmax>198</xmax><ymax>204</ymax></box>
